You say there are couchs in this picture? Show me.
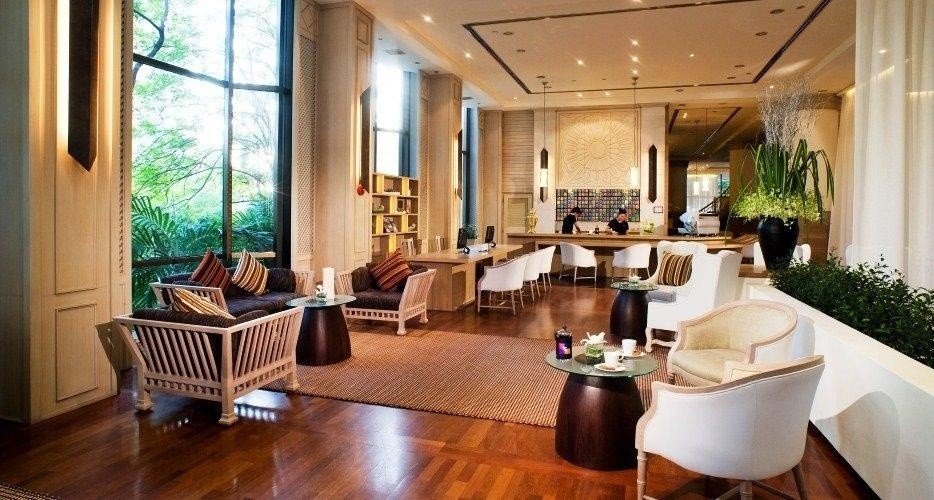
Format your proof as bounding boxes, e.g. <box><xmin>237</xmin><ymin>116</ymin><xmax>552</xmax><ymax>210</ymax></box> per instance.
<box><xmin>635</xmin><ymin>353</ymin><xmax>829</xmax><ymax>499</ymax></box>
<box><xmin>334</xmin><ymin>259</ymin><xmax>438</xmax><ymax>335</ymax></box>
<box><xmin>148</xmin><ymin>266</ymin><xmax>316</xmax><ymax>321</ymax></box>
<box><xmin>666</xmin><ymin>299</ymin><xmax>799</xmax><ymax>387</ymax></box>
<box><xmin>642</xmin><ymin>240</ymin><xmax>707</xmax><ymax>289</ymax></box>
<box><xmin>112</xmin><ymin>305</ymin><xmax>304</xmax><ymax>426</ymax></box>
<box><xmin>645</xmin><ymin>250</ymin><xmax>744</xmax><ymax>353</ymax></box>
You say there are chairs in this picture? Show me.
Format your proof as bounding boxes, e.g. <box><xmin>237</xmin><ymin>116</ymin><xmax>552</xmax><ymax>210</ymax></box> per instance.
<box><xmin>524</xmin><ymin>248</ymin><xmax>548</xmax><ymax>301</ymax></box>
<box><xmin>404</xmin><ymin>238</ymin><xmax>417</xmax><ymax>256</ymax></box>
<box><xmin>477</xmin><ymin>253</ymin><xmax>530</xmax><ymax>315</ymax></box>
<box><xmin>559</xmin><ymin>241</ymin><xmax>597</xmax><ymax>285</ymax></box>
<box><xmin>435</xmin><ymin>235</ymin><xmax>446</xmax><ymax>250</ymax></box>
<box><xmin>537</xmin><ymin>244</ymin><xmax>557</xmax><ymax>290</ymax></box>
<box><xmin>610</xmin><ymin>243</ymin><xmax>652</xmax><ymax>281</ymax></box>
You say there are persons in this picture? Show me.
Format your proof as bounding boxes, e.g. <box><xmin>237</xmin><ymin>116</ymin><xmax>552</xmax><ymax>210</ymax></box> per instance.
<box><xmin>606</xmin><ymin>209</ymin><xmax>630</xmax><ymax>235</ymax></box>
<box><xmin>562</xmin><ymin>207</ymin><xmax>584</xmax><ymax>234</ymax></box>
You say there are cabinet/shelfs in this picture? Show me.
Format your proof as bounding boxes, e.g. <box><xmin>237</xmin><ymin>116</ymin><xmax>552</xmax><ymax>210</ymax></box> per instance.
<box><xmin>371</xmin><ymin>172</ymin><xmax>421</xmax><ymax>257</ymax></box>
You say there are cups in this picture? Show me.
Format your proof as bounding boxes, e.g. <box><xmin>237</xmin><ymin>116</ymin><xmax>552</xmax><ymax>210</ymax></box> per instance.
<box><xmin>604</xmin><ymin>352</ymin><xmax>624</xmax><ymax>369</ymax></box>
<box><xmin>619</xmin><ymin>351</ymin><xmax>646</xmax><ymax>358</ymax></box>
<box><xmin>622</xmin><ymin>339</ymin><xmax>637</xmax><ymax>355</ymax></box>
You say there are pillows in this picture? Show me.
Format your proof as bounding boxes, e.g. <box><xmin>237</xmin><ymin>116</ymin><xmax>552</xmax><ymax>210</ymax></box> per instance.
<box><xmin>656</xmin><ymin>250</ymin><xmax>693</xmax><ymax>286</ymax></box>
<box><xmin>163</xmin><ymin>287</ymin><xmax>235</xmax><ymax>318</ymax></box>
<box><xmin>365</xmin><ymin>248</ymin><xmax>412</xmax><ymax>293</ymax></box>
<box><xmin>189</xmin><ymin>247</ymin><xmax>231</xmax><ymax>296</ymax></box>
<box><xmin>231</xmin><ymin>247</ymin><xmax>269</xmax><ymax>296</ymax></box>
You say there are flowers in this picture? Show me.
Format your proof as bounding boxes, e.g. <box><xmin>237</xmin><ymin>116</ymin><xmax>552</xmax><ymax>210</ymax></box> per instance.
<box><xmin>462</xmin><ymin>223</ymin><xmax>479</xmax><ymax>239</ymax></box>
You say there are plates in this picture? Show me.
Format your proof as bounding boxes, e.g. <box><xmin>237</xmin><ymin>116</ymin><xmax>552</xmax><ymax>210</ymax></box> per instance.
<box><xmin>594</xmin><ymin>362</ymin><xmax>625</xmax><ymax>372</ymax></box>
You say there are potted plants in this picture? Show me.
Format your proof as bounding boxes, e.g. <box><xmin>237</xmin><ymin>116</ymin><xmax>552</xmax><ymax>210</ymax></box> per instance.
<box><xmin>716</xmin><ymin>178</ymin><xmax>731</xmax><ymax>233</ymax></box>
<box><xmin>740</xmin><ymin>253</ymin><xmax>934</xmax><ymax>500</ymax></box>
<box><xmin>729</xmin><ymin>92</ymin><xmax>836</xmax><ymax>276</ymax></box>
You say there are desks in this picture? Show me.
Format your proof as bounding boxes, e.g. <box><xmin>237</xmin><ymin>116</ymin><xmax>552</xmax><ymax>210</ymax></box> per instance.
<box><xmin>407</xmin><ymin>243</ymin><xmax>523</xmax><ymax>311</ymax></box>
<box><xmin>504</xmin><ymin>233</ymin><xmax>731</xmax><ymax>281</ymax></box>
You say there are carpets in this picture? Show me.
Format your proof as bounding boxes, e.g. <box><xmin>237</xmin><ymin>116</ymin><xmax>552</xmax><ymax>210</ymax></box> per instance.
<box><xmin>254</xmin><ymin>323</ymin><xmax>693</xmax><ymax>437</ymax></box>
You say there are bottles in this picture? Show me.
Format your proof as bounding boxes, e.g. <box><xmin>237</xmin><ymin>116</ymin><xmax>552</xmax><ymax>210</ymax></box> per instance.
<box><xmin>594</xmin><ymin>226</ymin><xmax>599</xmax><ymax>236</ymax></box>
